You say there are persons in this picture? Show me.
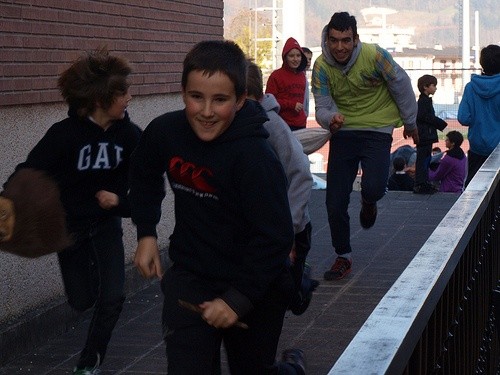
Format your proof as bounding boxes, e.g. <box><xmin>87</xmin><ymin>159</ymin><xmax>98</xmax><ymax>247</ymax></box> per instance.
<box><xmin>263</xmin><ymin>37</ymin><xmax>307</xmax><ymax>134</ymax></box>
<box><xmin>242</xmin><ymin>58</ymin><xmax>321</xmax><ymax>316</ymax></box>
<box><xmin>0</xmin><ymin>44</ymin><xmax>147</xmax><ymax>375</ymax></box>
<box><xmin>413</xmin><ymin>74</ymin><xmax>448</xmax><ymax>195</ymax></box>
<box><xmin>457</xmin><ymin>43</ymin><xmax>500</xmax><ymax>193</ymax></box>
<box><xmin>428</xmin><ymin>130</ymin><xmax>468</xmax><ymax>194</ymax></box>
<box><xmin>300</xmin><ymin>47</ymin><xmax>313</xmax><ymax>118</ymax></box>
<box><xmin>388</xmin><ymin>157</ymin><xmax>414</xmax><ymax>191</ymax></box>
<box><xmin>129</xmin><ymin>37</ymin><xmax>307</xmax><ymax>375</ymax></box>
<box><xmin>432</xmin><ymin>147</ymin><xmax>442</xmax><ymax>156</ymax></box>
<box><xmin>309</xmin><ymin>10</ymin><xmax>421</xmax><ymax>282</ymax></box>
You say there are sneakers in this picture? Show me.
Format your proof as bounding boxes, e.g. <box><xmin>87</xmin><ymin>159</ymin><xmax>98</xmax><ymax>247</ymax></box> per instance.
<box><xmin>323</xmin><ymin>256</ymin><xmax>353</xmax><ymax>281</ymax></box>
<box><xmin>360</xmin><ymin>197</ymin><xmax>378</xmax><ymax>229</ymax></box>
<box><xmin>292</xmin><ymin>258</ymin><xmax>313</xmax><ymax>315</ymax></box>
<box><xmin>282</xmin><ymin>348</ymin><xmax>308</xmax><ymax>373</ymax></box>
